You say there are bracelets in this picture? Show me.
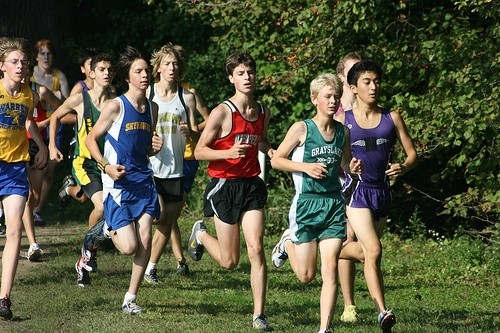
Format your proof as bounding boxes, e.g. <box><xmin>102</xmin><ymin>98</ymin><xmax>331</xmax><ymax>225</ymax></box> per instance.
<box><xmin>96</xmin><ymin>159</ymin><xmax>110</xmax><ymax>174</ymax></box>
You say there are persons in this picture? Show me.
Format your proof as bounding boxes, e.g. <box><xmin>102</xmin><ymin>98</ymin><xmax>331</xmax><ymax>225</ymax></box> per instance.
<box><xmin>48</xmin><ymin>52</ymin><xmax>120</xmax><ymax>288</ymax></box>
<box><xmin>144</xmin><ymin>42</ymin><xmax>201</xmax><ymax>289</ymax></box>
<box><xmin>271</xmin><ymin>73</ymin><xmax>367</xmax><ymax>333</ymax></box>
<box><xmin>338</xmin><ymin>60</ymin><xmax>416</xmax><ymax>333</ymax></box>
<box><xmin>0</xmin><ymin>39</ymin><xmax>70</xmax><ymax>261</ymax></box>
<box><xmin>0</xmin><ymin>36</ymin><xmax>49</xmax><ymax>322</ymax></box>
<box><xmin>59</xmin><ymin>49</ymin><xmax>119</xmax><ymax>204</ymax></box>
<box><xmin>83</xmin><ymin>46</ymin><xmax>164</xmax><ymax>315</ymax></box>
<box><xmin>170</xmin><ymin>43</ymin><xmax>209</xmax><ymax>278</ymax></box>
<box><xmin>188</xmin><ymin>53</ymin><xmax>277</xmax><ymax>331</ymax></box>
<box><xmin>334</xmin><ymin>52</ymin><xmax>385</xmax><ymax>325</ymax></box>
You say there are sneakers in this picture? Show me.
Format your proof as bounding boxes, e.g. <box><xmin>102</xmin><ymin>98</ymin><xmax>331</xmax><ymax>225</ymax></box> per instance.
<box><xmin>187</xmin><ymin>219</ymin><xmax>208</xmax><ymax>261</ymax></box>
<box><xmin>84</xmin><ymin>218</ymin><xmax>105</xmax><ymax>251</ymax></box>
<box><xmin>254</xmin><ymin>314</ymin><xmax>273</xmax><ymax>331</ymax></box>
<box><xmin>273</xmin><ymin>229</ymin><xmax>293</xmax><ymax>268</ymax></box>
<box><xmin>57</xmin><ymin>175</ymin><xmax>75</xmax><ymax>198</ymax></box>
<box><xmin>75</xmin><ymin>258</ymin><xmax>93</xmax><ymax>286</ymax></box>
<box><xmin>340</xmin><ymin>304</ymin><xmax>358</xmax><ymax>323</ymax></box>
<box><xmin>0</xmin><ymin>214</ymin><xmax>6</xmax><ymax>237</ymax></box>
<box><xmin>0</xmin><ymin>296</ymin><xmax>12</xmax><ymax>319</ymax></box>
<box><xmin>122</xmin><ymin>299</ymin><xmax>141</xmax><ymax>315</ymax></box>
<box><xmin>33</xmin><ymin>213</ymin><xmax>44</xmax><ymax>226</ymax></box>
<box><xmin>143</xmin><ymin>269</ymin><xmax>162</xmax><ymax>285</ymax></box>
<box><xmin>378</xmin><ymin>311</ymin><xmax>395</xmax><ymax>333</ymax></box>
<box><xmin>28</xmin><ymin>244</ymin><xmax>43</xmax><ymax>262</ymax></box>
<box><xmin>176</xmin><ymin>261</ymin><xmax>189</xmax><ymax>275</ymax></box>
<box><xmin>80</xmin><ymin>243</ymin><xmax>98</xmax><ymax>273</ymax></box>
